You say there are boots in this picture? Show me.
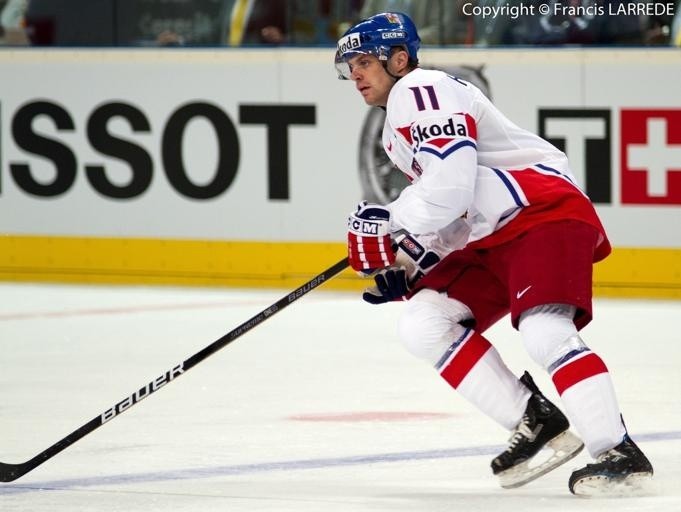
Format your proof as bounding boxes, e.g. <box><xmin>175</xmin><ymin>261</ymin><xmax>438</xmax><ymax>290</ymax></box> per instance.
<box><xmin>568</xmin><ymin>412</ymin><xmax>654</xmax><ymax>495</ymax></box>
<box><xmin>490</xmin><ymin>371</ymin><xmax>569</xmax><ymax>474</ymax></box>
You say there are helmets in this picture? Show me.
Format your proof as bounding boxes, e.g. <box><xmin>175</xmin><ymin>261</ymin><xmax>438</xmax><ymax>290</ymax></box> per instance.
<box><xmin>335</xmin><ymin>11</ymin><xmax>422</xmax><ymax>81</ymax></box>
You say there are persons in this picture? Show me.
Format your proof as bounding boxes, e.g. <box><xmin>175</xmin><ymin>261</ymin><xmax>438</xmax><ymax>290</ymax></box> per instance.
<box><xmin>334</xmin><ymin>11</ymin><xmax>656</xmax><ymax>494</ymax></box>
<box><xmin>2</xmin><ymin>0</ymin><xmax>681</xmax><ymax>47</ymax></box>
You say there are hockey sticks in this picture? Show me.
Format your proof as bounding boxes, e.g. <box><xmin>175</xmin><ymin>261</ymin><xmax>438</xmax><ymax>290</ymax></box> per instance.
<box><xmin>0</xmin><ymin>256</ymin><xmax>349</xmax><ymax>483</ymax></box>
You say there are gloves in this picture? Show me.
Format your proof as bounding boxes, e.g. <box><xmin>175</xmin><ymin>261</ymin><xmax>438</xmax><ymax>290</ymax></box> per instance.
<box><xmin>348</xmin><ymin>200</ymin><xmax>399</xmax><ymax>279</ymax></box>
<box><xmin>362</xmin><ymin>234</ymin><xmax>440</xmax><ymax>304</ymax></box>
